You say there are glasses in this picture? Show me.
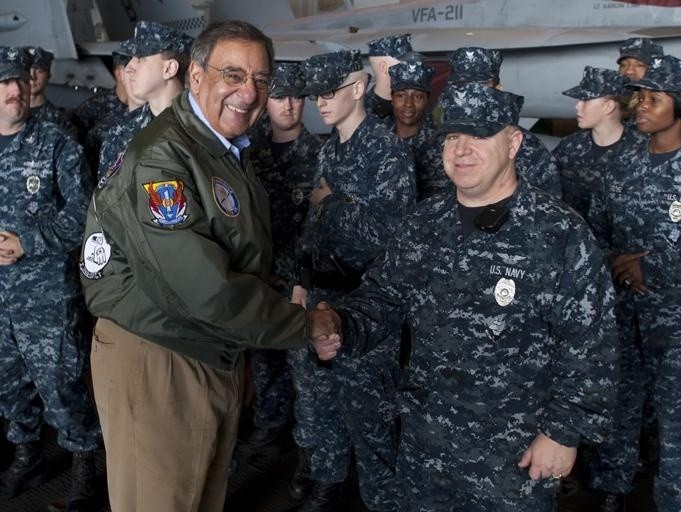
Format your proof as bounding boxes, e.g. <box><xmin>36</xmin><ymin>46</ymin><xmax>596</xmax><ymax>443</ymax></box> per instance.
<box><xmin>308</xmin><ymin>82</ymin><xmax>356</xmax><ymax>100</ymax></box>
<box><xmin>208</xmin><ymin>63</ymin><xmax>273</xmax><ymax>89</ymax></box>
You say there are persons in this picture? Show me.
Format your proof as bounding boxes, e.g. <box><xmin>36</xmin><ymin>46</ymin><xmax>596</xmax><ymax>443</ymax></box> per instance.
<box><xmin>2</xmin><ymin>17</ymin><xmax>681</xmax><ymax>511</ymax></box>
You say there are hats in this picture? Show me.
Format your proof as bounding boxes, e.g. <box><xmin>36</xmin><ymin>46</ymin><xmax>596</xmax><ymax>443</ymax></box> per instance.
<box><xmin>562</xmin><ymin>37</ymin><xmax>681</xmax><ymax>100</ymax></box>
<box><xmin>436</xmin><ymin>84</ymin><xmax>524</xmax><ymax>137</ymax></box>
<box><xmin>447</xmin><ymin>47</ymin><xmax>503</xmax><ymax>84</ymax></box>
<box><xmin>113</xmin><ymin>21</ymin><xmax>194</xmax><ymax>57</ymax></box>
<box><xmin>265</xmin><ymin>62</ymin><xmax>308</xmax><ymax>98</ymax></box>
<box><xmin>299</xmin><ymin>49</ymin><xmax>363</xmax><ymax>96</ymax></box>
<box><xmin>0</xmin><ymin>46</ymin><xmax>53</xmax><ymax>82</ymax></box>
<box><xmin>368</xmin><ymin>33</ymin><xmax>435</xmax><ymax>92</ymax></box>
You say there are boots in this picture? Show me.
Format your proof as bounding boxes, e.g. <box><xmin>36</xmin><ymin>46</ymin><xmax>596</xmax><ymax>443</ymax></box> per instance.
<box><xmin>48</xmin><ymin>450</ymin><xmax>106</xmax><ymax>512</ymax></box>
<box><xmin>1</xmin><ymin>443</ymin><xmax>47</xmax><ymax>502</ymax></box>
